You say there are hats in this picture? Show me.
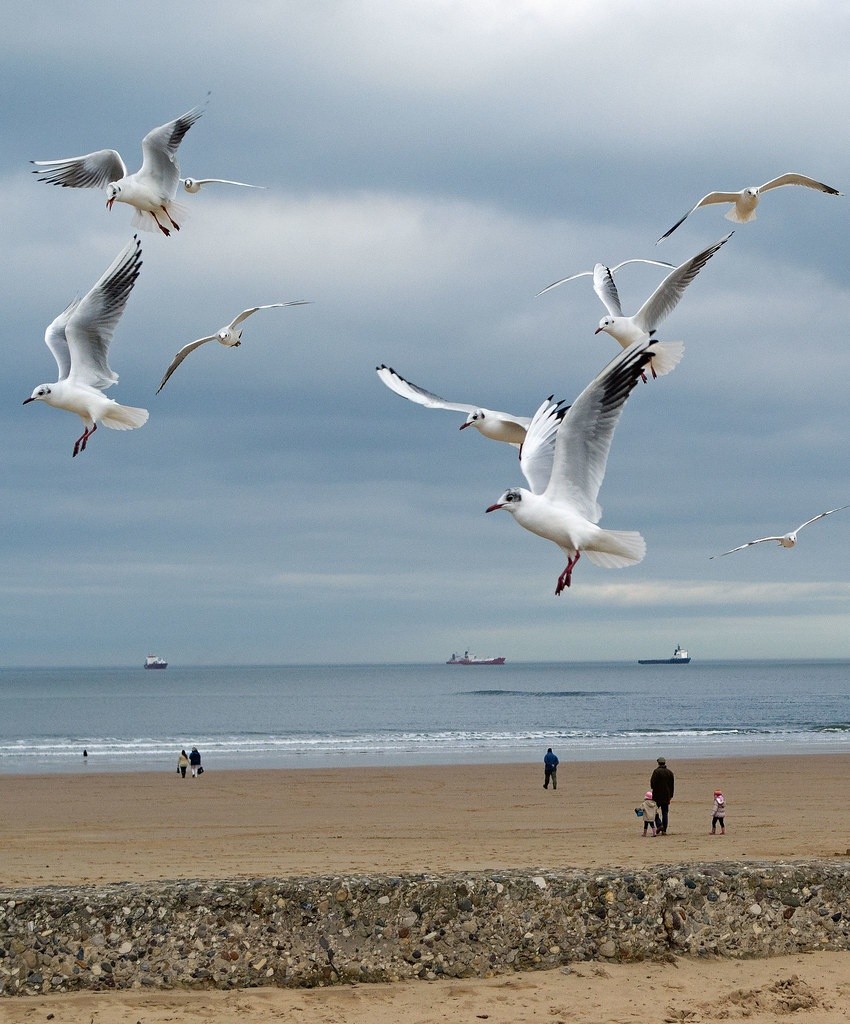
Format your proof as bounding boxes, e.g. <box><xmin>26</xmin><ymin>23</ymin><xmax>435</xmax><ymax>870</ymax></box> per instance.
<box><xmin>657</xmin><ymin>757</ymin><xmax>666</xmax><ymax>763</ymax></box>
<box><xmin>714</xmin><ymin>788</ymin><xmax>722</xmax><ymax>797</ymax></box>
<box><xmin>645</xmin><ymin>792</ymin><xmax>653</xmax><ymax>799</ymax></box>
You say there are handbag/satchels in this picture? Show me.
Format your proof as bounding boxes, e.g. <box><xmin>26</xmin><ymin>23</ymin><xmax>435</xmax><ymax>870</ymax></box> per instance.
<box><xmin>177</xmin><ymin>766</ymin><xmax>180</xmax><ymax>773</ymax></box>
<box><xmin>198</xmin><ymin>766</ymin><xmax>204</xmax><ymax>774</ymax></box>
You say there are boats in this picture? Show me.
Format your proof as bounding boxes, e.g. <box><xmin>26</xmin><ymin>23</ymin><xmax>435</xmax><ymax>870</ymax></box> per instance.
<box><xmin>143</xmin><ymin>651</ymin><xmax>169</xmax><ymax>670</ymax></box>
<box><xmin>446</xmin><ymin>649</ymin><xmax>506</xmax><ymax>666</ymax></box>
<box><xmin>637</xmin><ymin>643</ymin><xmax>691</xmax><ymax>665</ymax></box>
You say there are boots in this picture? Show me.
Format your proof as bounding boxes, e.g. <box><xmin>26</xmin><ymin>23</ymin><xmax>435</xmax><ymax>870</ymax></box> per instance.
<box><xmin>710</xmin><ymin>828</ymin><xmax>716</xmax><ymax>835</ymax></box>
<box><xmin>642</xmin><ymin>829</ymin><xmax>647</xmax><ymax>837</ymax></box>
<box><xmin>651</xmin><ymin>828</ymin><xmax>656</xmax><ymax>837</ymax></box>
<box><xmin>720</xmin><ymin>827</ymin><xmax>725</xmax><ymax>835</ymax></box>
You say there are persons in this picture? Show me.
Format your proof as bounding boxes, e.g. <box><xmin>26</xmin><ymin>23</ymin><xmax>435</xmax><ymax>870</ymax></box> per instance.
<box><xmin>542</xmin><ymin>748</ymin><xmax>560</xmax><ymax>790</ymax></box>
<box><xmin>709</xmin><ymin>790</ymin><xmax>726</xmax><ymax>835</ymax></box>
<box><xmin>650</xmin><ymin>757</ymin><xmax>674</xmax><ymax>838</ymax></box>
<box><xmin>638</xmin><ymin>792</ymin><xmax>657</xmax><ymax>837</ymax></box>
<box><xmin>84</xmin><ymin>749</ymin><xmax>88</xmax><ymax>762</ymax></box>
<box><xmin>177</xmin><ymin>747</ymin><xmax>204</xmax><ymax>778</ymax></box>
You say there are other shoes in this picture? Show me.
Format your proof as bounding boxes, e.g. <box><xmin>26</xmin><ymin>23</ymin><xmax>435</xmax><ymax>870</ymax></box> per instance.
<box><xmin>543</xmin><ymin>785</ymin><xmax>547</xmax><ymax>789</ymax></box>
<box><xmin>192</xmin><ymin>774</ymin><xmax>195</xmax><ymax>777</ymax></box>
<box><xmin>662</xmin><ymin>832</ymin><xmax>666</xmax><ymax>835</ymax></box>
<box><xmin>656</xmin><ymin>827</ymin><xmax>663</xmax><ymax>834</ymax></box>
<box><xmin>554</xmin><ymin>788</ymin><xmax>556</xmax><ymax>789</ymax></box>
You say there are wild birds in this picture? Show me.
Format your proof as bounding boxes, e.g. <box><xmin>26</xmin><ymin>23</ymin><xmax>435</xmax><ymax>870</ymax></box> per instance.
<box><xmin>156</xmin><ymin>301</ymin><xmax>313</xmax><ymax>395</ymax></box>
<box><xmin>22</xmin><ymin>234</ymin><xmax>150</xmax><ymax>458</ymax></box>
<box><xmin>655</xmin><ymin>172</ymin><xmax>846</xmax><ymax>247</ymax></box>
<box><xmin>535</xmin><ymin>230</ymin><xmax>735</xmax><ymax>384</ymax></box>
<box><xmin>535</xmin><ymin>258</ymin><xmax>678</xmax><ymax>298</ymax></box>
<box><xmin>177</xmin><ymin>178</ymin><xmax>267</xmax><ymax>194</ymax></box>
<box><xmin>29</xmin><ymin>92</ymin><xmax>212</xmax><ymax>238</ymax></box>
<box><xmin>374</xmin><ymin>364</ymin><xmax>534</xmax><ymax>448</ymax></box>
<box><xmin>485</xmin><ymin>328</ymin><xmax>659</xmax><ymax>597</ymax></box>
<box><xmin>708</xmin><ymin>504</ymin><xmax>850</xmax><ymax>560</ymax></box>
